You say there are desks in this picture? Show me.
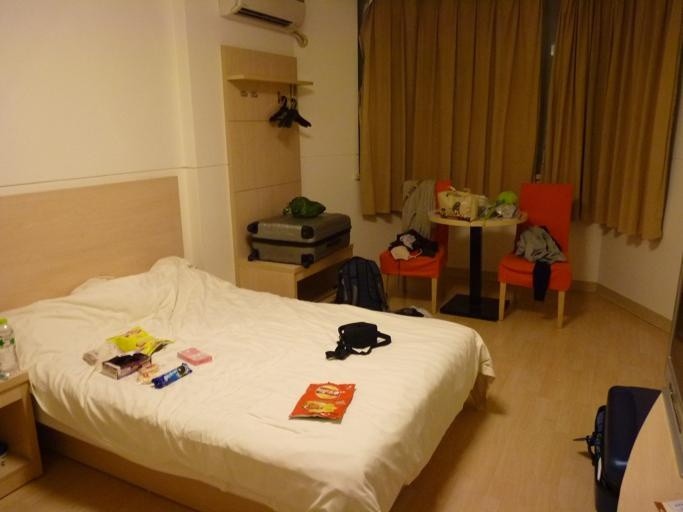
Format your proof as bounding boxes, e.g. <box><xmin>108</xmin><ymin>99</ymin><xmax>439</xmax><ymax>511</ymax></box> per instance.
<box><xmin>425</xmin><ymin>208</ymin><xmax>528</xmax><ymax>322</ymax></box>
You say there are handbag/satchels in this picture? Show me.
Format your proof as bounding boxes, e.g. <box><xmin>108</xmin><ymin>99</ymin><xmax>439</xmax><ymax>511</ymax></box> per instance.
<box><xmin>323</xmin><ymin>318</ymin><xmax>392</xmax><ymax>361</ymax></box>
<box><xmin>436</xmin><ymin>184</ymin><xmax>488</xmax><ymax>222</ymax></box>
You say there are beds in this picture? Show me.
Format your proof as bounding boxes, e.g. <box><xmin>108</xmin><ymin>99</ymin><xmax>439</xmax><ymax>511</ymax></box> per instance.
<box><xmin>0</xmin><ymin>174</ymin><xmax>482</xmax><ymax>511</ymax></box>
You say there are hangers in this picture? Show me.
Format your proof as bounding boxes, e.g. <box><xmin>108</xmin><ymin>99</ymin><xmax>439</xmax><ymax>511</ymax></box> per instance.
<box><xmin>267</xmin><ymin>96</ymin><xmax>311</xmax><ymax>129</ymax></box>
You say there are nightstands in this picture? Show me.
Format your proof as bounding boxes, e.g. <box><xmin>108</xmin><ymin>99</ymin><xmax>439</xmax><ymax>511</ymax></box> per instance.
<box><xmin>240</xmin><ymin>244</ymin><xmax>354</xmax><ymax>304</ymax></box>
<box><xmin>0</xmin><ymin>369</ymin><xmax>44</xmax><ymax>499</ymax></box>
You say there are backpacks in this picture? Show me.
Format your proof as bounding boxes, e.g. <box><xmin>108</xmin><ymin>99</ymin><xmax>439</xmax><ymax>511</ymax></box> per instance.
<box><xmin>333</xmin><ymin>251</ymin><xmax>390</xmax><ymax>313</ymax></box>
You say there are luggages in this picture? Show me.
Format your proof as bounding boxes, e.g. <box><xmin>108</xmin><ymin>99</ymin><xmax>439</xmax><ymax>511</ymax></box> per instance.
<box><xmin>590</xmin><ymin>382</ymin><xmax>666</xmax><ymax>512</ymax></box>
<box><xmin>246</xmin><ymin>210</ymin><xmax>352</xmax><ymax>269</ymax></box>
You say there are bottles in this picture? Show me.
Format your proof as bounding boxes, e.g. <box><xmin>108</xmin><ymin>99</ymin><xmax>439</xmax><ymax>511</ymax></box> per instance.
<box><xmin>0</xmin><ymin>318</ymin><xmax>19</xmax><ymax>380</ymax></box>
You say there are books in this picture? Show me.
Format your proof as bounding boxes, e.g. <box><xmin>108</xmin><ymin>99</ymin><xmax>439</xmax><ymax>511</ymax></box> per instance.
<box><xmin>287</xmin><ymin>383</ymin><xmax>359</xmax><ymax>422</ymax></box>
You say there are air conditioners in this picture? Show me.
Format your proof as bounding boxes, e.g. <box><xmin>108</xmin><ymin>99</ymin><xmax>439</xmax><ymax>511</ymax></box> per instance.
<box><xmin>217</xmin><ymin>0</ymin><xmax>306</xmax><ymax>36</ymax></box>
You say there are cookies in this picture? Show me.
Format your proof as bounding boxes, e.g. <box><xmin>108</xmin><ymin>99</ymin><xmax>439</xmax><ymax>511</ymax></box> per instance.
<box><xmin>177</xmin><ymin>365</ymin><xmax>185</xmax><ymax>374</ymax></box>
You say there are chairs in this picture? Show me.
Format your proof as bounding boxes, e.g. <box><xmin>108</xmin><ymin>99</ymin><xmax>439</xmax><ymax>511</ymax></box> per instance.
<box><xmin>498</xmin><ymin>183</ymin><xmax>573</xmax><ymax>330</ymax></box>
<box><xmin>378</xmin><ymin>176</ymin><xmax>451</xmax><ymax>313</ymax></box>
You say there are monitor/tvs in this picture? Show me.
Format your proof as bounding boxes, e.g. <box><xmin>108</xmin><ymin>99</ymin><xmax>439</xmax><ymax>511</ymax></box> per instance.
<box><xmin>662</xmin><ymin>252</ymin><xmax>683</xmax><ymax>479</ymax></box>
